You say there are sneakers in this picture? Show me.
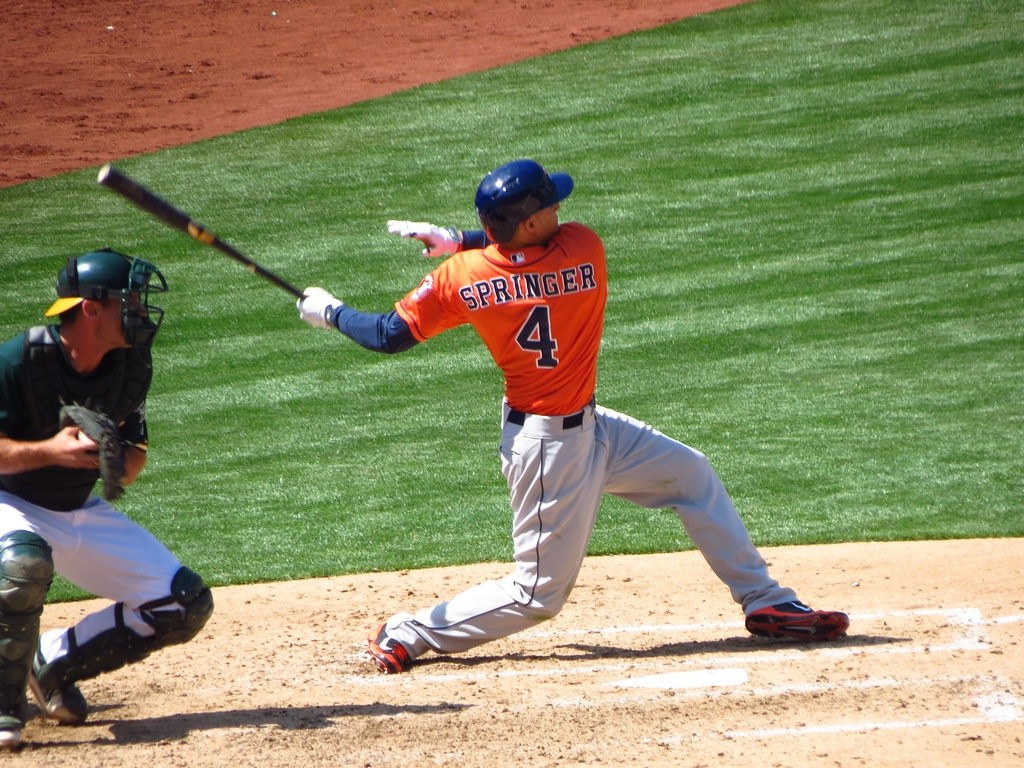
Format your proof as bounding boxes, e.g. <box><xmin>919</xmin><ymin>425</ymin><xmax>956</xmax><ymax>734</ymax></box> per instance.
<box><xmin>25</xmin><ymin>636</ymin><xmax>88</xmax><ymax>726</ymax></box>
<box><xmin>745</xmin><ymin>599</ymin><xmax>849</xmax><ymax>641</ymax></box>
<box><xmin>365</xmin><ymin>624</ymin><xmax>411</xmax><ymax>675</ymax></box>
<box><xmin>0</xmin><ymin>703</ymin><xmax>23</xmax><ymax>747</ymax></box>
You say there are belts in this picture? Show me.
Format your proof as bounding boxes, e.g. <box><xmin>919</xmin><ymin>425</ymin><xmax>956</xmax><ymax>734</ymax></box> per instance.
<box><xmin>506</xmin><ymin>394</ymin><xmax>596</xmax><ymax>430</ymax></box>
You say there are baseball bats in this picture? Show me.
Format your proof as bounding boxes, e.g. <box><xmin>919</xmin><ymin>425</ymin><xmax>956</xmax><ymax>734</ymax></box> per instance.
<box><xmin>98</xmin><ymin>163</ymin><xmax>306</xmax><ymax>299</ymax></box>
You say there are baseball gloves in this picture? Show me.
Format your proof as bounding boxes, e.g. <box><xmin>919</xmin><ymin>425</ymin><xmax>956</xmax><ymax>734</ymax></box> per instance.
<box><xmin>55</xmin><ymin>400</ymin><xmax>130</xmax><ymax>504</ymax></box>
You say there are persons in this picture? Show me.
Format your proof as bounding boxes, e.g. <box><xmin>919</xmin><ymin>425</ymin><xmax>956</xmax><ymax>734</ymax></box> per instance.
<box><xmin>296</xmin><ymin>161</ymin><xmax>850</xmax><ymax>674</ymax></box>
<box><xmin>1</xmin><ymin>247</ymin><xmax>214</xmax><ymax>749</ymax></box>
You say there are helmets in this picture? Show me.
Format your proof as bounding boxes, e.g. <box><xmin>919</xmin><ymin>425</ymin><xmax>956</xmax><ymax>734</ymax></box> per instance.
<box><xmin>43</xmin><ymin>248</ymin><xmax>134</xmax><ymax>318</ymax></box>
<box><xmin>472</xmin><ymin>159</ymin><xmax>575</xmax><ymax>224</ymax></box>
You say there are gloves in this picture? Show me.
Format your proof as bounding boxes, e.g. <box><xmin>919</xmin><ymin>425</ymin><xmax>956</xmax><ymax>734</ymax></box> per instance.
<box><xmin>295</xmin><ymin>284</ymin><xmax>343</xmax><ymax>331</ymax></box>
<box><xmin>387</xmin><ymin>218</ymin><xmax>461</xmax><ymax>258</ymax></box>
